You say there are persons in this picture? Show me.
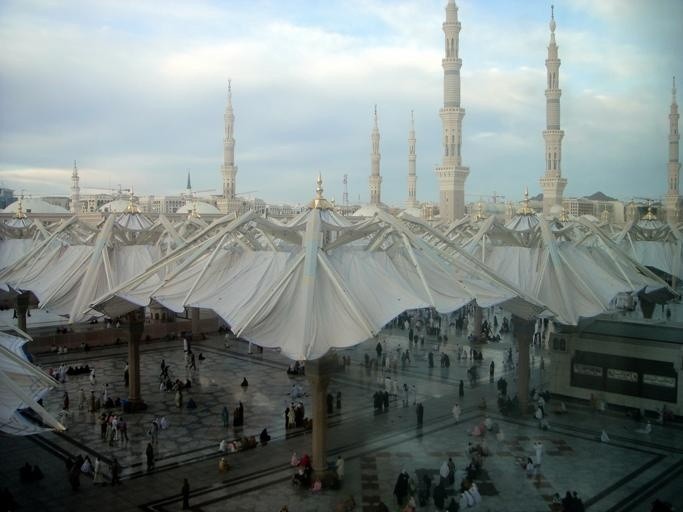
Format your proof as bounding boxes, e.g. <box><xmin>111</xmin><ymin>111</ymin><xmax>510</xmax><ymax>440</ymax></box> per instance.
<box><xmin>10</xmin><ymin>297</ymin><xmax>670</xmax><ymax>512</ymax></box>
